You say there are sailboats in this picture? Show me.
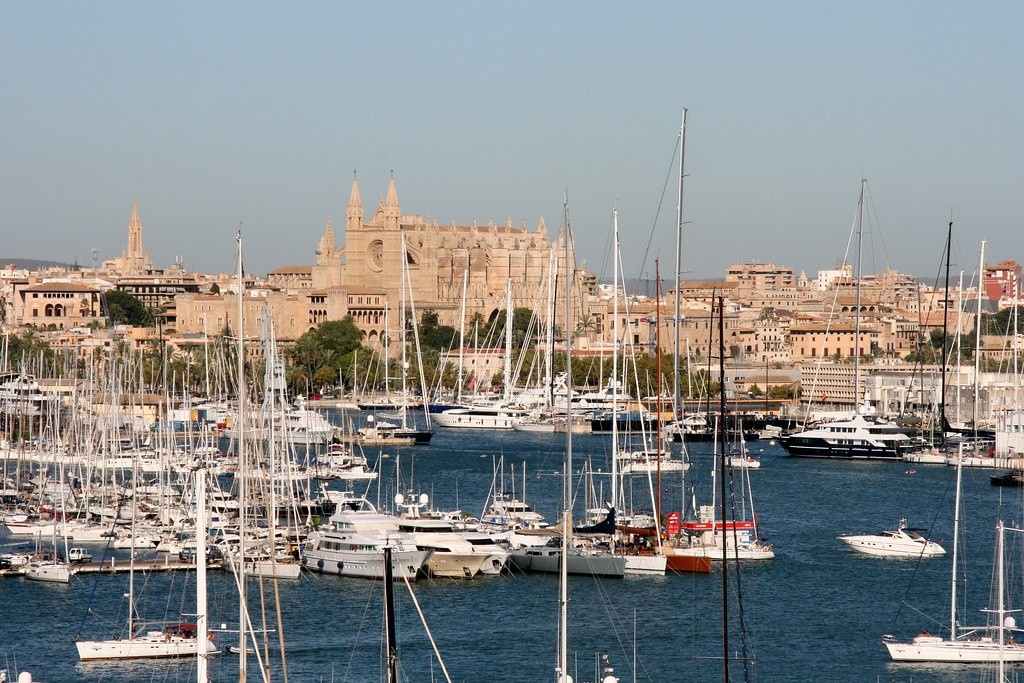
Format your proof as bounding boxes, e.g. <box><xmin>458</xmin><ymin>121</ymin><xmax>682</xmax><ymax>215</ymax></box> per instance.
<box><xmin>1</xmin><ymin>107</ymin><xmax>1024</xmax><ymax>683</ymax></box>
